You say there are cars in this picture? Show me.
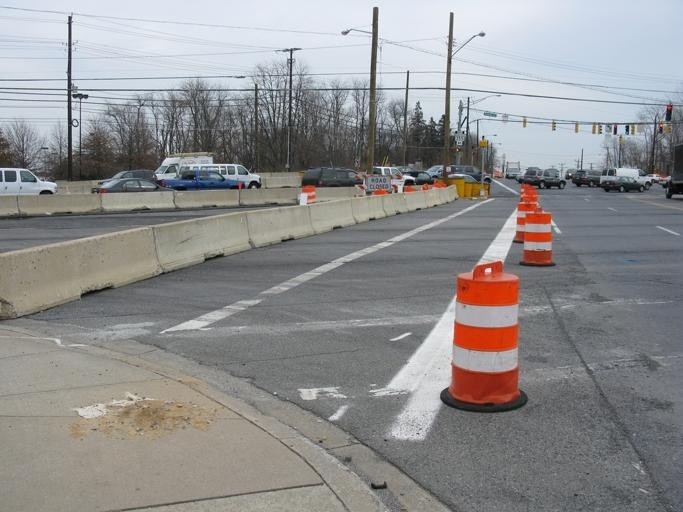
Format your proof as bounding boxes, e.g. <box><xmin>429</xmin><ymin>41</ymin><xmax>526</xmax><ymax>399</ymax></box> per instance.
<box><xmin>517</xmin><ymin>167</ymin><xmax>567</xmax><ymax>189</ymax></box>
<box><xmin>659</xmin><ymin>176</ymin><xmax>672</xmax><ymax>188</ymax></box>
<box><xmin>1</xmin><ymin>168</ymin><xmax>57</xmax><ymax>195</ymax></box>
<box><xmin>91</xmin><ymin>178</ymin><xmax>174</xmax><ymax>193</ymax></box>
<box><xmin>566</xmin><ymin>168</ymin><xmax>659</xmax><ymax>192</ymax></box>
<box><xmin>98</xmin><ymin>170</ymin><xmax>154</xmax><ymax>185</ymax></box>
<box><xmin>364</xmin><ymin>164</ymin><xmax>491</xmax><ymax>194</ymax></box>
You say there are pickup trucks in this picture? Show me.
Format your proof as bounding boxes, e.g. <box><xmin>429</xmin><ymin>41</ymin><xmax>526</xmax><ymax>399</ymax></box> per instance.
<box><xmin>156</xmin><ymin>170</ymin><xmax>240</xmax><ymax>190</ymax></box>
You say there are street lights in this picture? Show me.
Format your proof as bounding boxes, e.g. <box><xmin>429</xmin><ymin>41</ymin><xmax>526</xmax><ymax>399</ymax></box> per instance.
<box><xmin>342</xmin><ymin>29</ymin><xmax>378</xmax><ymax>176</ymax></box>
<box><xmin>443</xmin><ymin>32</ymin><xmax>502</xmax><ymax>186</ymax></box>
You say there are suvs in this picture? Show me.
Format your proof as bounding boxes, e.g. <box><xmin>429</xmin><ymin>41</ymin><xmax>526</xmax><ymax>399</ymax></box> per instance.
<box><xmin>302</xmin><ymin>167</ymin><xmax>363</xmax><ymax>187</ymax></box>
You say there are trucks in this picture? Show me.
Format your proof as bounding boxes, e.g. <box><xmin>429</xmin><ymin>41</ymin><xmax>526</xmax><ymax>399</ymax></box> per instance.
<box><xmin>506</xmin><ymin>162</ymin><xmax>521</xmax><ymax>178</ymax></box>
<box><xmin>662</xmin><ymin>144</ymin><xmax>683</xmax><ymax>199</ymax></box>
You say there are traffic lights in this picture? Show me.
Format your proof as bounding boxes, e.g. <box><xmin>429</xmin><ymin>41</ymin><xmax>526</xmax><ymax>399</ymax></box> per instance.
<box><xmin>597</xmin><ymin>124</ymin><xmax>602</xmax><ymax>134</ymax></box>
<box><xmin>614</xmin><ymin>125</ymin><xmax>617</xmax><ymax>135</ymax></box>
<box><xmin>625</xmin><ymin>126</ymin><xmax>629</xmax><ymax>135</ymax></box>
<box><xmin>666</xmin><ymin>105</ymin><xmax>672</xmax><ymax>121</ymax></box>
<box><xmin>659</xmin><ymin>124</ymin><xmax>663</xmax><ymax>134</ymax></box>
<box><xmin>551</xmin><ymin>122</ymin><xmax>557</xmax><ymax>132</ymax></box>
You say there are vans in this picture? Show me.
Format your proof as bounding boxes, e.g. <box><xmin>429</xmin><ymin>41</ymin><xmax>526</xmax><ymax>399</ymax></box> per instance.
<box><xmin>153</xmin><ymin>152</ymin><xmax>213</xmax><ymax>184</ymax></box>
<box><xmin>179</xmin><ymin>164</ymin><xmax>261</xmax><ymax>189</ymax></box>
